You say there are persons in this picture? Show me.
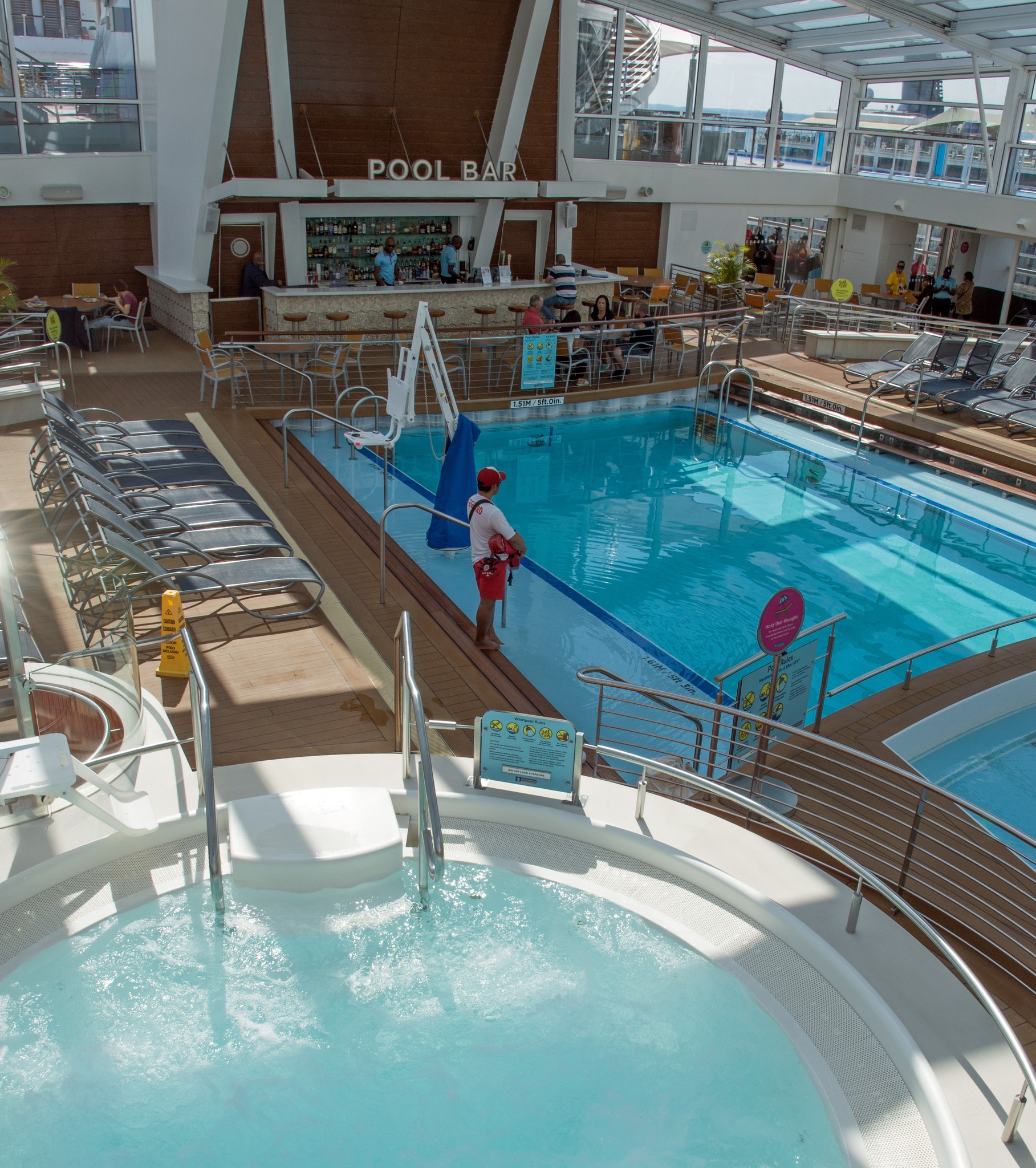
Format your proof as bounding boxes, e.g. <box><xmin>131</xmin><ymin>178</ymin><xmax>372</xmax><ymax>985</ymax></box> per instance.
<box><xmin>83</xmin><ymin>279</ymin><xmax>139</xmax><ymax>330</ymax></box>
<box><xmin>951</xmin><ymin>271</ymin><xmax>974</xmax><ymax>332</ymax></box>
<box><xmin>811</xmin><ymin>133</ymin><xmax>816</xmax><ymax>143</ymax></box>
<box><xmin>781</xmin><ymin>130</ymin><xmax>786</xmax><ymax>139</ymax></box>
<box><xmin>606</xmin><ymin>302</ymin><xmax>655</xmax><ymax>380</ymax></box>
<box><xmin>437</xmin><ymin>234</ymin><xmax>467</xmax><ymax>284</ymax></box>
<box><xmin>911</xmin><ymin>275</ymin><xmax>936</xmax><ymax>332</ymax></box>
<box><xmin>929</xmin><ymin>267</ymin><xmax>957</xmax><ymax>332</ymax></box>
<box><xmin>466</xmin><ymin>465</ymin><xmax>528</xmax><ymax>652</ymax></box>
<box><xmin>765</xmin><ymin>98</ymin><xmax>784</xmax><ymax>167</ymax></box>
<box><xmin>580</xmin><ymin>294</ymin><xmax>617</xmax><ymax>373</ymax></box>
<box><xmin>241</xmin><ymin>251</ymin><xmax>284</xmax><ymax>347</ymax></box>
<box><xmin>522</xmin><ymin>294</ymin><xmax>559</xmax><ymax>336</ymax></box>
<box><xmin>796</xmin><ymin>133</ymin><xmax>800</xmax><ymax>141</ymax></box>
<box><xmin>884</xmin><ymin>261</ymin><xmax>911</xmax><ymax>328</ymax></box>
<box><xmin>905</xmin><ymin>254</ymin><xmax>928</xmax><ymax>311</ymax></box>
<box><xmin>556</xmin><ymin>310</ymin><xmax>590</xmax><ymax>386</ymax></box>
<box><xmin>374</xmin><ymin>237</ymin><xmax>405</xmax><ymax>287</ymax></box>
<box><xmin>745</xmin><ymin>223</ymin><xmax>826</xmax><ymax>283</ymax></box>
<box><xmin>539</xmin><ymin>254</ymin><xmax>577</xmax><ymax>324</ymax></box>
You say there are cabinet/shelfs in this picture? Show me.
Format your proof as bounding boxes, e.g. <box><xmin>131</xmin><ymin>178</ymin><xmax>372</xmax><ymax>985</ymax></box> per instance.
<box><xmin>306</xmin><ymin>216</ymin><xmax>461</xmax><ymax>284</ymax></box>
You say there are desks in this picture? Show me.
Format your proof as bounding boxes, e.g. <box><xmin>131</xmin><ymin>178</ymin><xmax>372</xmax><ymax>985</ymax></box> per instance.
<box><xmin>254</xmin><ymin>339</ymin><xmax>316</xmax><ymax>402</ymax></box>
<box><xmin>16</xmin><ymin>296</ymin><xmax>109</xmax><ymax>351</ymax></box>
<box><xmin>865</xmin><ymin>293</ymin><xmax>902</xmax><ymax>331</ymax></box>
<box><xmin>614</xmin><ymin>276</ymin><xmax>676</xmax><ymax>317</ymax></box>
<box><xmin>449</xmin><ymin>334</ymin><xmax>508</xmax><ymax>393</ymax></box>
<box><xmin>579</xmin><ymin>330</ymin><xmax>632</xmax><ymax>385</ymax></box>
<box><xmin>666</xmin><ymin>317</ymin><xmax>719</xmax><ymax>375</ymax></box>
<box><xmin>738</xmin><ymin>283</ymin><xmax>764</xmax><ymax>309</ymax></box>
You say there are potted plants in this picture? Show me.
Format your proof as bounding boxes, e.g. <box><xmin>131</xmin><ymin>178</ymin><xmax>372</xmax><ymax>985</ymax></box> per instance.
<box><xmin>88</xmin><ymin>25</ymin><xmax>96</xmax><ymax>40</ymax></box>
<box><xmin>704</xmin><ymin>238</ymin><xmax>758</xmax><ymax>326</ymax></box>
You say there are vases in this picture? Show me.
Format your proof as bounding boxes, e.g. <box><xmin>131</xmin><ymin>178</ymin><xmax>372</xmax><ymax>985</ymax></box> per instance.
<box><xmin>83</xmin><ymin>35</ymin><xmax>88</xmax><ymax>39</ymax></box>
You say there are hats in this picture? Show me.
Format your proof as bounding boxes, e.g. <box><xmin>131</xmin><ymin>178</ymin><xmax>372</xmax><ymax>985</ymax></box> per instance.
<box><xmin>774</xmin><ymin>227</ymin><xmax>782</xmax><ymax>232</ymax></box>
<box><xmin>800</xmin><ymin>235</ymin><xmax>809</xmax><ymax>240</ymax></box>
<box><xmin>897</xmin><ymin>261</ymin><xmax>905</xmax><ymax>268</ymax></box>
<box><xmin>944</xmin><ymin>267</ymin><xmax>952</xmax><ymax>275</ymax></box>
<box><xmin>477</xmin><ymin>466</ymin><xmax>507</xmax><ymax>487</ymax></box>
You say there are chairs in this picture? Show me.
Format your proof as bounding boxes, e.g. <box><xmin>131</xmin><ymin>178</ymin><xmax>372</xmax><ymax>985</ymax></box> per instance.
<box><xmin>497</xmin><ymin>266</ymin><xmax>1036</xmax><ymax>430</ymax></box>
<box><xmin>0</xmin><ymin>283</ymin><xmax>365</xmax><ymax>659</ymax></box>
<box><xmin>67</xmin><ymin>25</ymin><xmax>88</xmax><ymax>40</ymax></box>
<box><xmin>396</xmin><ymin>334</ymin><xmax>467</xmax><ymax>402</ymax></box>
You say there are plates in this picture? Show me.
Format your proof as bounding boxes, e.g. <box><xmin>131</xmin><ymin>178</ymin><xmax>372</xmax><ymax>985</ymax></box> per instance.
<box><xmin>82</xmin><ymin>297</ymin><xmax>98</xmax><ymax>300</ymax></box>
<box><xmin>76</xmin><ymin>296</ymin><xmax>90</xmax><ymax>298</ymax></box>
<box><xmin>32</xmin><ymin>304</ymin><xmax>50</xmax><ymax>307</ymax></box>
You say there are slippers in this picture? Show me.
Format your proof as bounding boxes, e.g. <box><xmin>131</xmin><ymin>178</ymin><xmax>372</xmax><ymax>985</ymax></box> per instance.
<box><xmin>576</xmin><ymin>380</ymin><xmax>589</xmax><ymax>386</ymax></box>
<box><xmin>563</xmin><ymin>378</ymin><xmax>571</xmax><ymax>382</ymax></box>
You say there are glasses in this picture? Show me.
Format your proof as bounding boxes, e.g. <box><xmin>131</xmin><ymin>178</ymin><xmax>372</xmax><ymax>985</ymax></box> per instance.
<box><xmin>386</xmin><ymin>243</ymin><xmax>396</xmax><ymax>248</ymax></box>
<box><xmin>457</xmin><ymin>241</ymin><xmax>463</xmax><ymax>246</ymax></box>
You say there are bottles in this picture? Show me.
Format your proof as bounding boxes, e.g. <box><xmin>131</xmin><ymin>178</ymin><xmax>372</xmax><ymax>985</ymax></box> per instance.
<box><xmin>307</xmin><ymin>236</ymin><xmax>451</xmax><ymax>258</ymax></box>
<box><xmin>308</xmin><ymin>258</ymin><xmax>441</xmax><ymax>283</ymax></box>
<box><xmin>307</xmin><ymin>216</ymin><xmax>452</xmax><ymax>236</ymax></box>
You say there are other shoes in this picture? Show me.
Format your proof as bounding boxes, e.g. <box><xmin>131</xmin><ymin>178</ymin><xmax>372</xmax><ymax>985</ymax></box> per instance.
<box><xmin>607</xmin><ymin>369</ymin><xmax>630</xmax><ymax>379</ymax></box>
<box><xmin>557</xmin><ymin>370</ymin><xmax>564</xmax><ymax>374</ymax></box>
<box><xmin>600</xmin><ymin>364</ymin><xmax>610</xmax><ymax>373</ymax></box>
<box><xmin>554</xmin><ymin>320</ymin><xmax>560</xmax><ymax>329</ymax></box>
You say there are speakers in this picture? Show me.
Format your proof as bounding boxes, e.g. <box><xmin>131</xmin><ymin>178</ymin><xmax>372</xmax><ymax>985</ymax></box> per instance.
<box><xmin>564</xmin><ymin>204</ymin><xmax>577</xmax><ymax>229</ymax></box>
<box><xmin>203</xmin><ymin>206</ymin><xmax>220</xmax><ymax>234</ymax></box>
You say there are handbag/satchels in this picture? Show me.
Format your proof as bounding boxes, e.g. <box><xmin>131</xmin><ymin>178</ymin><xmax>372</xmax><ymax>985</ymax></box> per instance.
<box><xmin>950</xmin><ymin>302</ymin><xmax>955</xmax><ymax>311</ymax></box>
<box><xmin>110</xmin><ymin>310</ymin><xmax>125</xmax><ymax>321</ymax></box>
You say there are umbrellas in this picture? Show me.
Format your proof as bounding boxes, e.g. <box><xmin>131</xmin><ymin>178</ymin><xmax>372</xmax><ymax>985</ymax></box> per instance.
<box><xmin>903</xmin><ymin>106</ymin><xmax>1036</xmax><ymax>140</ymax></box>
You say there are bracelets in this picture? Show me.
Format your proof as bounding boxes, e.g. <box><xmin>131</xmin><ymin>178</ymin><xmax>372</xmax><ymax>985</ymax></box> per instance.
<box><xmin>108</xmin><ymin>297</ymin><xmax>110</xmax><ymax>301</ymax></box>
<box><xmin>547</xmin><ymin>327</ymin><xmax>551</xmax><ymax>332</ymax></box>
<box><xmin>626</xmin><ymin>324</ymin><xmax>629</xmax><ymax>328</ymax></box>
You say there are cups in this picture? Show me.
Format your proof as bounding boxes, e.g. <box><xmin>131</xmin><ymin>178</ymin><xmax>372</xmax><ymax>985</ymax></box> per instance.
<box><xmin>899</xmin><ymin>290</ymin><xmax>903</xmax><ymax>297</ymax></box>
<box><xmin>582</xmin><ymin>269</ymin><xmax>587</xmax><ymax>276</ymax></box>
<box><xmin>399</xmin><ymin>280</ymin><xmax>405</xmax><ymax>285</ymax></box>
<box><xmin>539</xmin><ymin>273</ymin><xmax>544</xmax><ymax>281</ymax></box>
<box><xmin>610</xmin><ymin>324</ymin><xmax>616</xmax><ymax>329</ymax></box>
<box><xmin>890</xmin><ymin>288</ymin><xmax>894</xmax><ymax>294</ymax></box>
<box><xmin>602</xmin><ymin>324</ymin><xmax>607</xmax><ymax>330</ymax></box>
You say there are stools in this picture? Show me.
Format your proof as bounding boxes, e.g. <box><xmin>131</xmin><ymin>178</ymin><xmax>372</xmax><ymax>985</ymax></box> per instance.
<box><xmin>284</xmin><ymin>294</ymin><xmax>640</xmax><ymax>362</ymax></box>
